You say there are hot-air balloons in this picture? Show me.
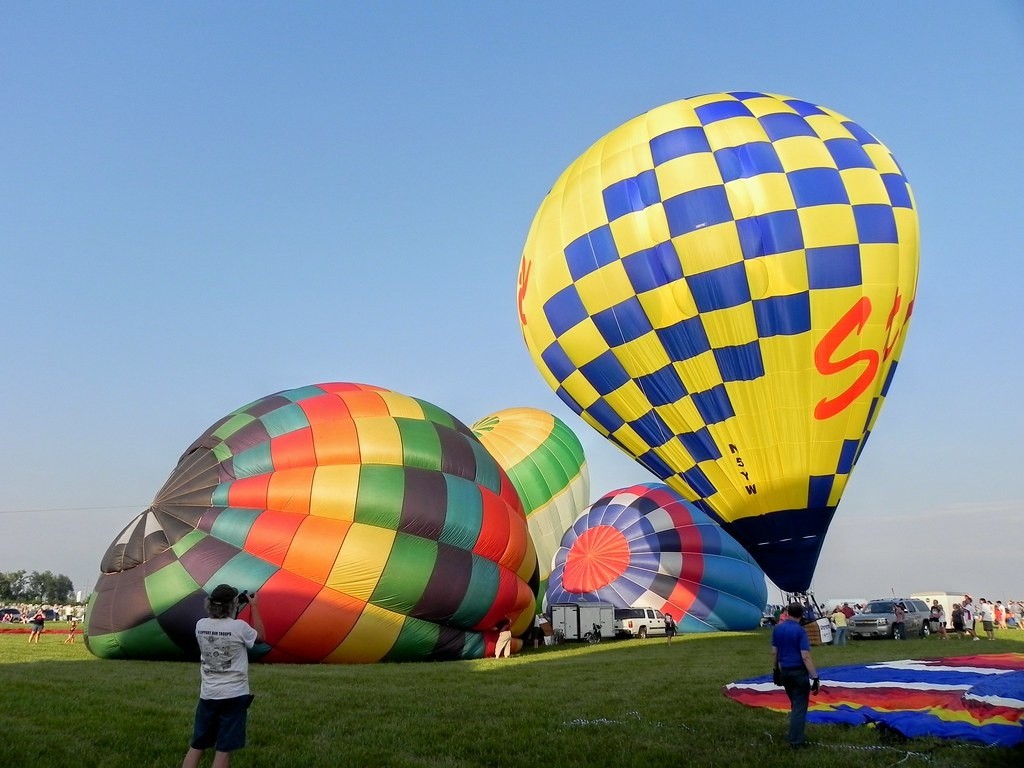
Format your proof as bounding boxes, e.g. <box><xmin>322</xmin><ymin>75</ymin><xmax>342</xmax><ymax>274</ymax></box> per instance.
<box><xmin>517</xmin><ymin>91</ymin><xmax>919</xmax><ymax>645</ymax></box>
<box><xmin>468</xmin><ymin>408</ymin><xmax>590</xmax><ymax>636</ymax></box>
<box><xmin>84</xmin><ymin>383</ymin><xmax>544</xmax><ymax>664</ymax></box>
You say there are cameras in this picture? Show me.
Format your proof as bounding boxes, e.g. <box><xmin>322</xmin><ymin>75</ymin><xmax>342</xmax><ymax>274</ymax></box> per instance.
<box><xmin>237</xmin><ymin>590</ymin><xmax>254</xmax><ymax>604</ymax></box>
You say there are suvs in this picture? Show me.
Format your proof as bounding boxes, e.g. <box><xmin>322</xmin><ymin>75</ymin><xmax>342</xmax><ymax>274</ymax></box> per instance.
<box><xmin>22</xmin><ymin>609</ymin><xmax>58</xmax><ymax>622</ymax></box>
<box><xmin>615</xmin><ymin>608</ymin><xmax>678</xmax><ymax>639</ymax></box>
<box><xmin>848</xmin><ymin>598</ymin><xmax>931</xmax><ymax>639</ymax></box>
<box><xmin>0</xmin><ymin>609</ymin><xmax>21</xmax><ymax>621</ymax></box>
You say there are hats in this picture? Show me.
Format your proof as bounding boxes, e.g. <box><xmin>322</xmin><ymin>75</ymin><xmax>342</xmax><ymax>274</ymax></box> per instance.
<box><xmin>844</xmin><ymin>602</ymin><xmax>848</xmax><ymax>605</ymax></box>
<box><xmin>838</xmin><ymin>606</ymin><xmax>842</xmax><ymax>610</ymax></box>
<box><xmin>208</xmin><ymin>584</ymin><xmax>238</xmax><ymax>606</ymax></box>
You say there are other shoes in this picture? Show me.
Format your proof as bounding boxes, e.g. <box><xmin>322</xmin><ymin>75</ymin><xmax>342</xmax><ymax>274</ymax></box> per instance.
<box><xmin>988</xmin><ymin>637</ymin><xmax>995</xmax><ymax>641</ymax></box>
<box><xmin>972</xmin><ymin>637</ymin><xmax>980</xmax><ymax>641</ymax></box>
<box><xmin>790</xmin><ymin>737</ymin><xmax>809</xmax><ymax>750</ymax></box>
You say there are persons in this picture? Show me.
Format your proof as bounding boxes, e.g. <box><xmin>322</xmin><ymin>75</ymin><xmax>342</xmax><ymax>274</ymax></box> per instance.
<box><xmin>854</xmin><ymin>604</ymin><xmax>863</xmax><ymax>614</ymax></box>
<box><xmin>493</xmin><ymin>615</ymin><xmax>512</xmax><ymax>658</ymax></box>
<box><xmin>69</xmin><ymin>618</ymin><xmax>78</xmax><ymax>643</ymax></box>
<box><xmin>664</xmin><ymin>613</ymin><xmax>675</xmax><ymax>645</ymax></box>
<box><xmin>893</xmin><ymin>605</ymin><xmax>906</xmax><ymax>640</ymax></box>
<box><xmin>842</xmin><ymin>602</ymin><xmax>855</xmax><ymax>619</ymax></box>
<box><xmin>3</xmin><ymin>613</ymin><xmax>13</xmax><ymax>623</ymax></box>
<box><xmin>930</xmin><ymin>600</ymin><xmax>949</xmax><ymax>639</ymax></box>
<box><xmin>19</xmin><ymin>606</ymin><xmax>29</xmax><ymax>624</ymax></box>
<box><xmin>53</xmin><ymin>603</ymin><xmax>73</xmax><ymax>623</ymax></box>
<box><xmin>775</xmin><ymin>606</ymin><xmax>782</xmax><ymax>621</ymax></box>
<box><xmin>779</xmin><ymin>608</ymin><xmax>788</xmax><ymax>623</ymax></box>
<box><xmin>770</xmin><ymin>602</ymin><xmax>820</xmax><ymax>746</ymax></box>
<box><xmin>533</xmin><ymin>615</ymin><xmax>540</xmax><ymax>648</ymax></box>
<box><xmin>950</xmin><ymin>594</ymin><xmax>1024</xmax><ymax>641</ymax></box>
<box><xmin>831</xmin><ymin>606</ymin><xmax>847</xmax><ymax>645</ymax></box>
<box><xmin>803</xmin><ymin>602</ymin><xmax>825</xmax><ymax>618</ymax></box>
<box><xmin>28</xmin><ymin>608</ymin><xmax>46</xmax><ymax>643</ymax></box>
<box><xmin>181</xmin><ymin>584</ymin><xmax>265</xmax><ymax>768</ymax></box>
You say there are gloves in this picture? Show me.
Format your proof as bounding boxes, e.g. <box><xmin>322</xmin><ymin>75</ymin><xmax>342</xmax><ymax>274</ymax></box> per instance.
<box><xmin>812</xmin><ymin>676</ymin><xmax>820</xmax><ymax>696</ymax></box>
<box><xmin>773</xmin><ymin>668</ymin><xmax>783</xmax><ymax>686</ymax></box>
